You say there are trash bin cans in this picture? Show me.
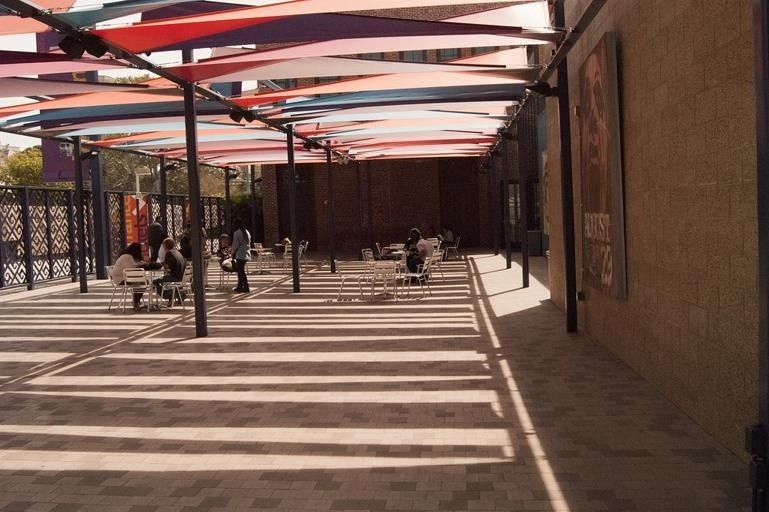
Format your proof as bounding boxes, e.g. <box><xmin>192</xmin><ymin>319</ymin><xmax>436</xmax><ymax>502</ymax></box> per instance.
<box><xmin>528</xmin><ymin>231</ymin><xmax>542</xmax><ymax>256</ymax></box>
<box><xmin>2</xmin><ymin>240</ymin><xmax>18</xmax><ymax>263</ymax></box>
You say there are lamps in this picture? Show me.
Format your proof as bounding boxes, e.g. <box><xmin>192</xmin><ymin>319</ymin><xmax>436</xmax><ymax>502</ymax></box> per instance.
<box><xmin>303</xmin><ymin>139</ymin><xmax>322</xmax><ymax>150</ymax></box>
<box><xmin>81</xmin><ymin>148</ymin><xmax>100</xmax><ymax>163</ymax></box>
<box><xmin>229</xmin><ymin>105</ymin><xmax>257</xmax><ymax>125</ymax></box>
<box><xmin>519</xmin><ymin>81</ymin><xmax>560</xmax><ymax>100</ymax></box>
<box><xmin>56</xmin><ymin>30</ymin><xmax>110</xmax><ymax>60</ymax></box>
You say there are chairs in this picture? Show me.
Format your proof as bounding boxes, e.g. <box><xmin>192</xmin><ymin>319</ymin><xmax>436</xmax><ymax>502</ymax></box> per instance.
<box><xmin>333</xmin><ymin>234</ymin><xmax>462</xmax><ymax>303</ymax></box>
<box><xmin>104</xmin><ymin>236</ymin><xmax>313</xmax><ymax>316</ymax></box>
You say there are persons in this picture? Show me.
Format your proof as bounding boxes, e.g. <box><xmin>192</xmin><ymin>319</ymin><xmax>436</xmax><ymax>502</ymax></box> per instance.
<box><xmin>153</xmin><ymin>237</ymin><xmax>187</xmax><ymax>306</ymax></box>
<box><xmin>147</xmin><ymin>215</ymin><xmax>164</xmax><ymax>264</ymax></box>
<box><xmin>406</xmin><ymin>228</ymin><xmax>433</xmax><ymax>283</ymax></box>
<box><xmin>439</xmin><ymin>225</ymin><xmax>454</xmax><ymax>262</ymax></box>
<box><xmin>230</xmin><ymin>217</ymin><xmax>252</xmax><ymax>292</ymax></box>
<box><xmin>111</xmin><ymin>242</ymin><xmax>148</xmax><ymax>312</ymax></box>
